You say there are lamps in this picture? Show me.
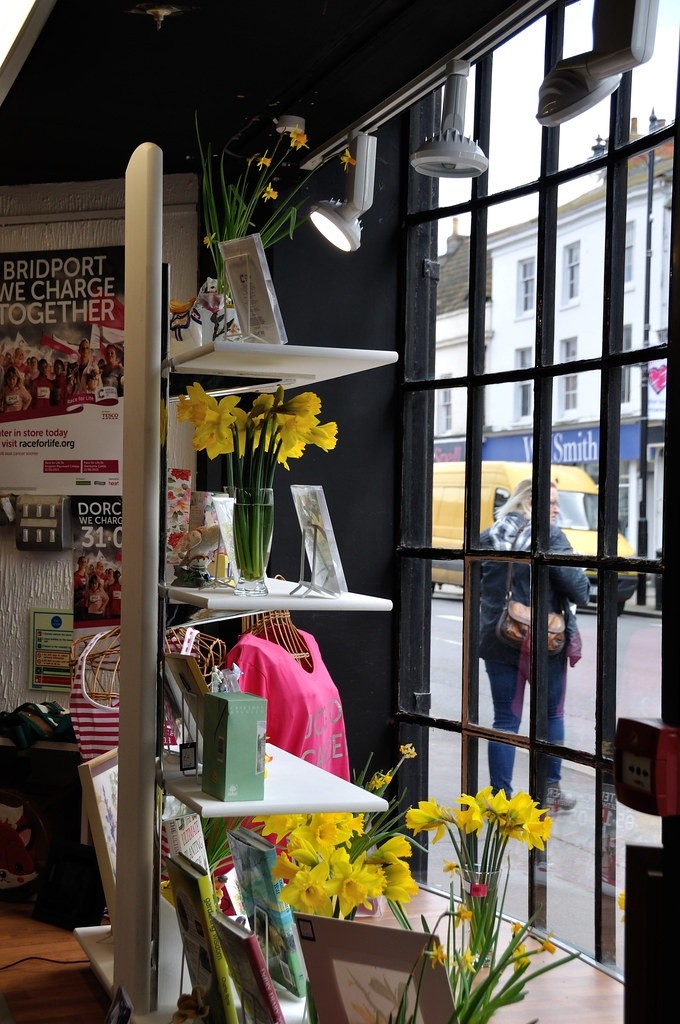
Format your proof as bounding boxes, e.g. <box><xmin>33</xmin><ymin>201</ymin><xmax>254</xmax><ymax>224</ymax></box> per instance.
<box><xmin>409</xmin><ymin>60</ymin><xmax>488</xmax><ymax>179</ymax></box>
<box><xmin>535</xmin><ymin>0</ymin><xmax>659</xmax><ymax>128</ymax></box>
<box><xmin>308</xmin><ymin>132</ymin><xmax>377</xmax><ymax>252</ymax></box>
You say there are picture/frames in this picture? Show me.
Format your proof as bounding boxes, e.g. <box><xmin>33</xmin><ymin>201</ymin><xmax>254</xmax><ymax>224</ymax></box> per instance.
<box><xmin>293</xmin><ymin>912</ymin><xmax>454</xmax><ymax>1024</ymax></box>
<box><xmin>164</xmin><ymin>654</ymin><xmax>211</xmax><ymax>783</ymax></box>
<box><xmin>217</xmin><ymin>233</ymin><xmax>288</xmax><ymax>345</ymax></box>
<box><xmin>288</xmin><ymin>484</ymin><xmax>350</xmax><ymax>599</ymax></box>
<box><xmin>78</xmin><ymin>745</ymin><xmax>119</xmax><ymax>940</ymax></box>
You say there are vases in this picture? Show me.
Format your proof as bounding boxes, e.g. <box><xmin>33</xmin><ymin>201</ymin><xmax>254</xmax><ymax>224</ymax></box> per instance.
<box><xmin>456</xmin><ymin>864</ymin><xmax>502</xmax><ymax>966</ymax></box>
<box><xmin>210</xmin><ymin>494</ymin><xmax>237</xmax><ymax>584</ymax></box>
<box><xmin>188</xmin><ymin>306</ymin><xmax>242</xmax><ymax>348</ymax></box>
<box><xmin>233</xmin><ymin>487</ymin><xmax>275</xmax><ymax>598</ymax></box>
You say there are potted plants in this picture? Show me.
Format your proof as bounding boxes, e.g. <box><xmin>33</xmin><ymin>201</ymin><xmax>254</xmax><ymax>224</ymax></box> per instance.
<box><xmin>160</xmin><ymin>809</ymin><xmax>289</xmax><ymax>910</ymax></box>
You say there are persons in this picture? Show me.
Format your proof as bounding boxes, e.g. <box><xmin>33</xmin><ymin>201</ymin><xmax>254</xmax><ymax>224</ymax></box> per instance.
<box><xmin>473</xmin><ymin>482</ymin><xmax>590</xmax><ymax>814</ymax></box>
<box><xmin>0</xmin><ymin>339</ymin><xmax>124</xmax><ymax>414</ymax></box>
<box><xmin>74</xmin><ymin>558</ymin><xmax>125</xmax><ymax>621</ymax></box>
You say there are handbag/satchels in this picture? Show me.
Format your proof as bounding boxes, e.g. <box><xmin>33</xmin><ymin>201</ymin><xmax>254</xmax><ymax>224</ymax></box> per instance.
<box><xmin>495</xmin><ymin>600</ymin><xmax>566</xmax><ymax>656</ymax></box>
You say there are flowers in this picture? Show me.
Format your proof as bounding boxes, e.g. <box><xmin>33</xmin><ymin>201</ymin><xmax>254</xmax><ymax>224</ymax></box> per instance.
<box><xmin>379</xmin><ymin>895</ymin><xmax>582</xmax><ymax>1024</ymax></box>
<box><xmin>192</xmin><ymin>110</ymin><xmax>357</xmax><ymax>304</ymax></box>
<box><xmin>405</xmin><ymin>786</ymin><xmax>554</xmax><ymax>952</ymax></box>
<box><xmin>176</xmin><ymin>381</ymin><xmax>338</xmax><ymax>580</ymax></box>
<box><xmin>249</xmin><ymin>742</ymin><xmax>429</xmax><ymax>920</ymax></box>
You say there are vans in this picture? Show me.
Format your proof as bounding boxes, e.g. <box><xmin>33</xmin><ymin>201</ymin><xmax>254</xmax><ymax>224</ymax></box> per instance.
<box><xmin>431</xmin><ymin>459</ymin><xmax>640</xmax><ymax>617</ymax></box>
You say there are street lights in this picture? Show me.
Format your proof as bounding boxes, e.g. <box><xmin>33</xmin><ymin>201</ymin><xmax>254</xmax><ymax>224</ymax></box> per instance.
<box><xmin>584</xmin><ymin>108</ymin><xmax>659</xmax><ymax>605</ymax></box>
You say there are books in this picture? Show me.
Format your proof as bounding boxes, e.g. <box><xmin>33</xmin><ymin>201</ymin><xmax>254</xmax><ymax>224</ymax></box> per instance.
<box><xmin>165</xmin><ymin>827</ymin><xmax>307</xmax><ymax>1024</ymax></box>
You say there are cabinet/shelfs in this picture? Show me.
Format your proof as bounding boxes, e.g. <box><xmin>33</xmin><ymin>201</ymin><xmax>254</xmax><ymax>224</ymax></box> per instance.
<box><xmin>73</xmin><ymin>143</ymin><xmax>393</xmax><ymax>1024</ymax></box>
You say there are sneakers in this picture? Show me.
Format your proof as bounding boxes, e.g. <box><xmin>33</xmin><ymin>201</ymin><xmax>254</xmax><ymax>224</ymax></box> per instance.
<box><xmin>546</xmin><ymin>782</ymin><xmax>576</xmax><ymax>810</ymax></box>
